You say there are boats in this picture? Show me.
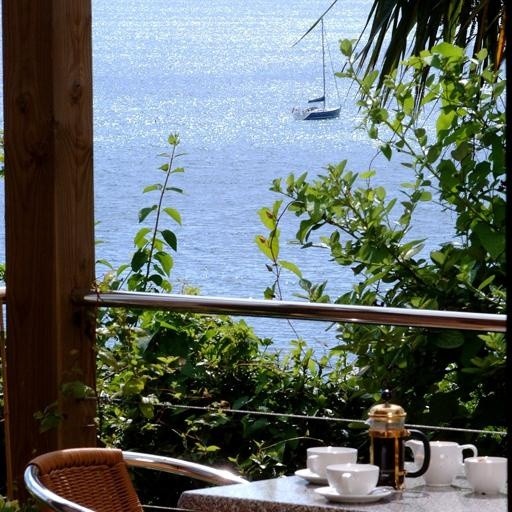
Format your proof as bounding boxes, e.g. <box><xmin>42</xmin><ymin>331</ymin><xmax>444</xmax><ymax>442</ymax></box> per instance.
<box><xmin>291</xmin><ymin>103</ymin><xmax>342</xmax><ymax>121</ymax></box>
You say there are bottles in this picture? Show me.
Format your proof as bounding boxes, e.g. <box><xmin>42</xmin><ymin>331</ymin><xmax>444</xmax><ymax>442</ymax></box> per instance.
<box><xmin>364</xmin><ymin>388</ymin><xmax>431</xmax><ymax>493</ymax></box>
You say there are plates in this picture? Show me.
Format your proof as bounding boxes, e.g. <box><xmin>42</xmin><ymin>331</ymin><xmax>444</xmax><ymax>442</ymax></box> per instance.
<box><xmin>313</xmin><ymin>485</ymin><xmax>392</xmax><ymax>504</ymax></box>
<box><xmin>294</xmin><ymin>467</ymin><xmax>331</xmax><ymax>485</ymax></box>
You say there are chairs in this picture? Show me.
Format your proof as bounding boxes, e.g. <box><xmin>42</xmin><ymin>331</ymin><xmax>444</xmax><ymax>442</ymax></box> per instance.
<box><xmin>17</xmin><ymin>446</ymin><xmax>251</xmax><ymax>511</ymax></box>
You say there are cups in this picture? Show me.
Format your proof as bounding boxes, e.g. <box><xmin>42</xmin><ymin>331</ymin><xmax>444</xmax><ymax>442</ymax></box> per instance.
<box><xmin>306</xmin><ymin>445</ymin><xmax>359</xmax><ymax>477</ymax></box>
<box><xmin>462</xmin><ymin>454</ymin><xmax>508</xmax><ymax>497</ymax></box>
<box><xmin>325</xmin><ymin>463</ymin><xmax>381</xmax><ymax>495</ymax></box>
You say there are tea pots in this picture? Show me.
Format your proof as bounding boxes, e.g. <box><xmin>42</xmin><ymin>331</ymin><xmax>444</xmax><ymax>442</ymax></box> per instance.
<box><xmin>405</xmin><ymin>439</ymin><xmax>478</xmax><ymax>488</ymax></box>
